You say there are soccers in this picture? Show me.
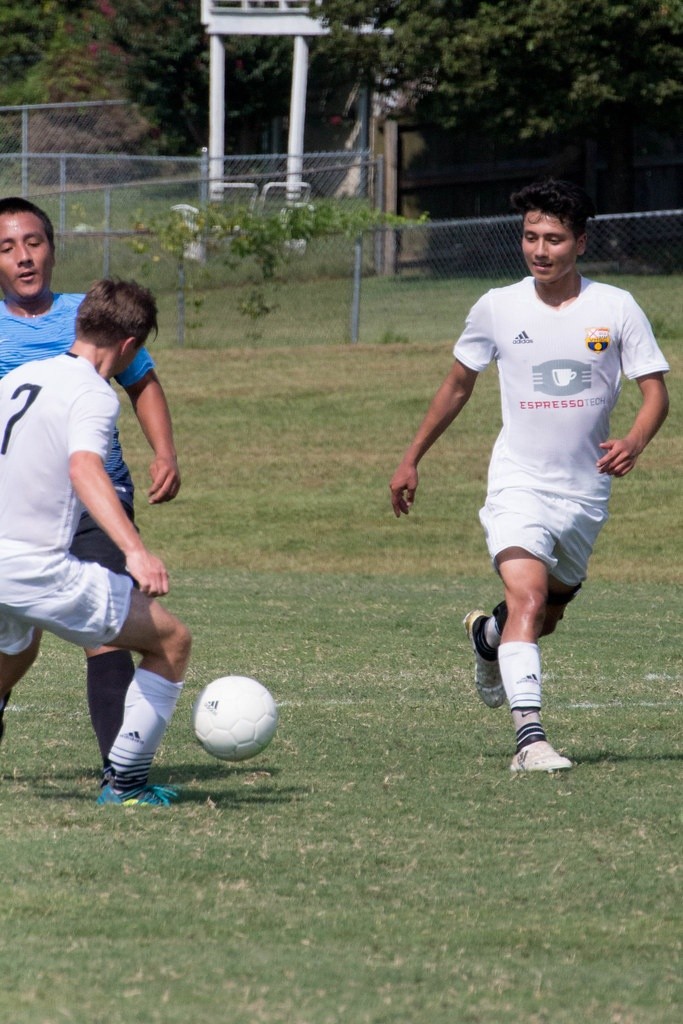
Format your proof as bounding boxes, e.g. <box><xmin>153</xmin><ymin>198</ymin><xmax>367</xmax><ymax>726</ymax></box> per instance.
<box><xmin>192</xmin><ymin>675</ymin><xmax>279</xmax><ymax>761</ymax></box>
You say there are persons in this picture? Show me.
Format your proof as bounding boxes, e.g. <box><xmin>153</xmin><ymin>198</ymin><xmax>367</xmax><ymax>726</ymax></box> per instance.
<box><xmin>0</xmin><ymin>197</ymin><xmax>192</xmax><ymax>806</ymax></box>
<box><xmin>390</xmin><ymin>178</ymin><xmax>670</xmax><ymax>771</ymax></box>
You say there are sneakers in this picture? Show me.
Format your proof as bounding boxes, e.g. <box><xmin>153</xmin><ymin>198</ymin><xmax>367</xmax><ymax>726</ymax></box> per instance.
<box><xmin>95</xmin><ymin>780</ymin><xmax>178</xmax><ymax>808</ymax></box>
<box><xmin>510</xmin><ymin>744</ymin><xmax>573</xmax><ymax>772</ymax></box>
<box><xmin>462</xmin><ymin>609</ymin><xmax>506</xmax><ymax>709</ymax></box>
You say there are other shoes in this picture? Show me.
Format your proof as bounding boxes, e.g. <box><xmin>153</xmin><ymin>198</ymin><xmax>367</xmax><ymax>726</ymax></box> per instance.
<box><xmin>100</xmin><ymin>767</ymin><xmax>113</xmax><ymax>788</ymax></box>
